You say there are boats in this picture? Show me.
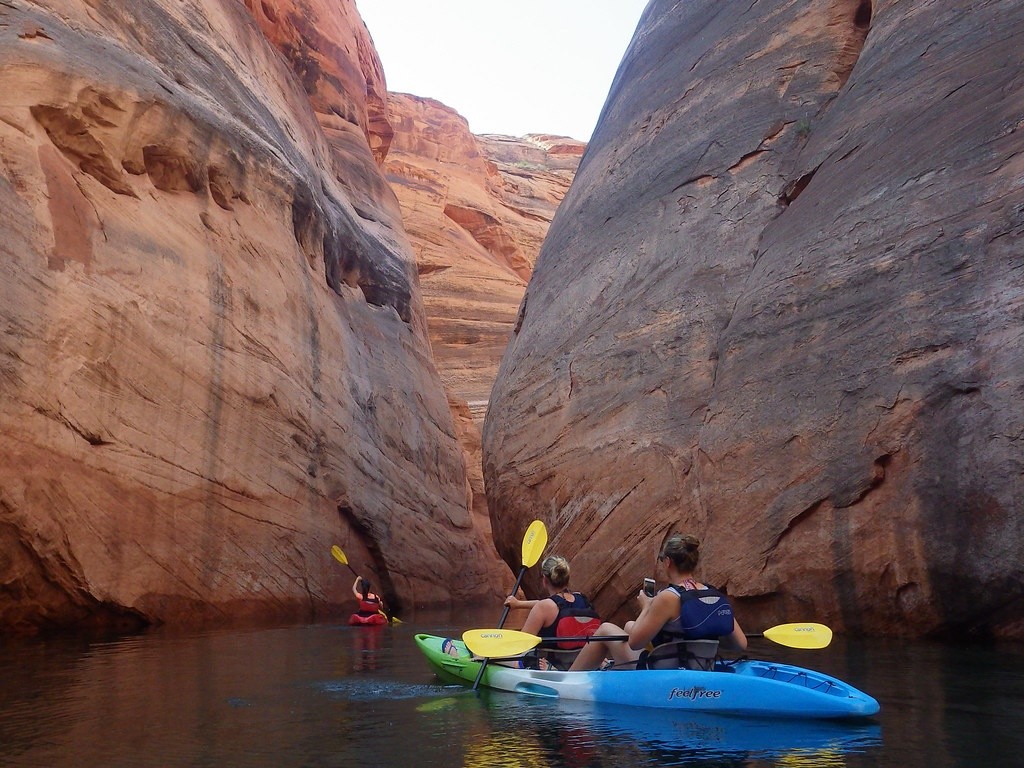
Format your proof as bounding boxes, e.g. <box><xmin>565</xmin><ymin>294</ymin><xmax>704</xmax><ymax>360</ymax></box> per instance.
<box><xmin>347</xmin><ymin>615</ymin><xmax>388</xmax><ymax>628</ymax></box>
<box><xmin>413</xmin><ymin>632</ymin><xmax>882</xmax><ymax>717</ymax></box>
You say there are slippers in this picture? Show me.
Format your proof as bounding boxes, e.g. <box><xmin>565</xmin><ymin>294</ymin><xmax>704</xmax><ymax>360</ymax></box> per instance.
<box><xmin>537</xmin><ymin>657</ymin><xmax>558</xmax><ymax>671</ymax></box>
<box><xmin>442</xmin><ymin>636</ymin><xmax>457</xmax><ymax>657</ymax></box>
<box><xmin>600</xmin><ymin>657</ymin><xmax>613</xmax><ymax>670</ymax></box>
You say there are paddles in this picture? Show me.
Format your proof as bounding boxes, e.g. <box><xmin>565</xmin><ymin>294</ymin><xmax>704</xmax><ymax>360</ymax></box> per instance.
<box><xmin>330</xmin><ymin>544</ymin><xmax>403</xmax><ymax>623</ymax></box>
<box><xmin>468</xmin><ymin>519</ymin><xmax>550</xmax><ymax>691</ymax></box>
<box><xmin>459</xmin><ymin>621</ymin><xmax>835</xmax><ymax>659</ymax></box>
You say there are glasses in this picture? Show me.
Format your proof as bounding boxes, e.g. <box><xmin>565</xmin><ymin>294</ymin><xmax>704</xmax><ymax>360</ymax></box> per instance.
<box><xmin>659</xmin><ymin>556</ymin><xmax>666</xmax><ymax>562</ymax></box>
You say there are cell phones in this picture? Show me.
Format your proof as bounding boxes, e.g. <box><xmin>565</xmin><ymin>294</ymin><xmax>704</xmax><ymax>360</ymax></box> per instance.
<box><xmin>645</xmin><ymin>579</ymin><xmax>655</xmax><ymax>599</ymax></box>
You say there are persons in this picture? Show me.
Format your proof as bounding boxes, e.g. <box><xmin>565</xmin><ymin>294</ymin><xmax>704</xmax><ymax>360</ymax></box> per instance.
<box><xmin>539</xmin><ymin>535</ymin><xmax>748</xmax><ymax>671</ymax></box>
<box><xmin>352</xmin><ymin>576</ymin><xmax>384</xmax><ymax>616</ymax></box>
<box><xmin>442</xmin><ymin>556</ymin><xmax>597</xmax><ymax>667</ymax></box>
